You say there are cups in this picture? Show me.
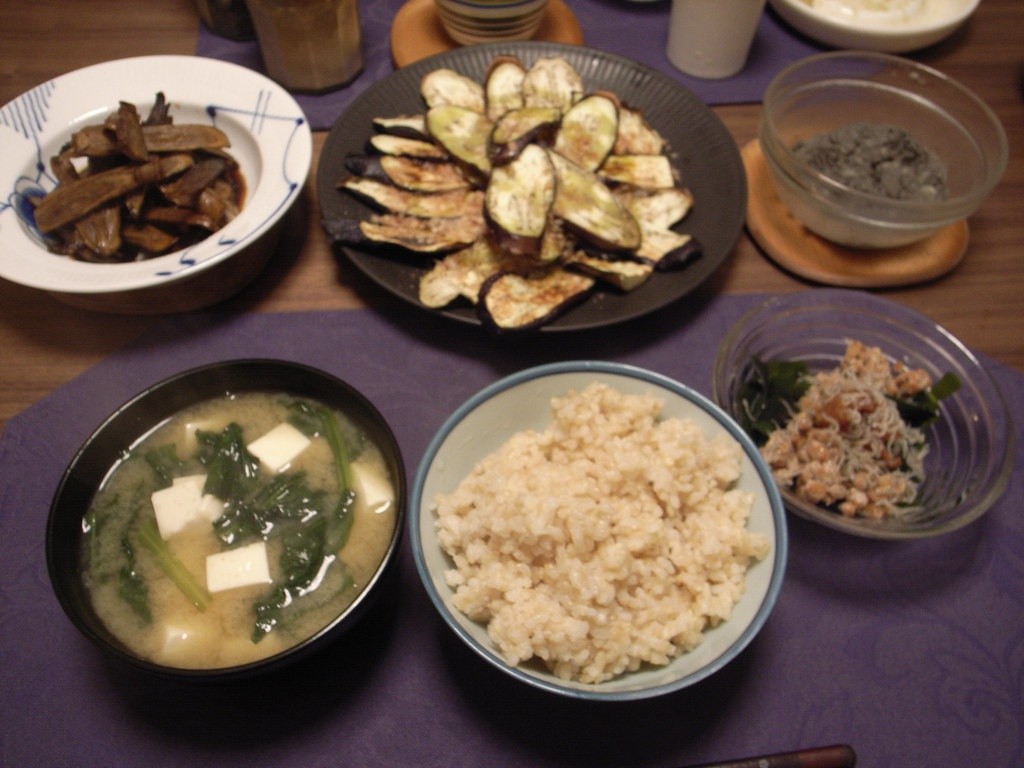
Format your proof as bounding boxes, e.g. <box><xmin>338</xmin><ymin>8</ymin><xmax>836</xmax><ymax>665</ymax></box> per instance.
<box><xmin>192</xmin><ymin>1</ymin><xmax>255</xmax><ymax>40</ymax></box>
<box><xmin>666</xmin><ymin>0</ymin><xmax>769</xmax><ymax>81</ymax></box>
<box><xmin>437</xmin><ymin>0</ymin><xmax>544</xmax><ymax>45</ymax></box>
<box><xmin>244</xmin><ymin>0</ymin><xmax>364</xmax><ymax>95</ymax></box>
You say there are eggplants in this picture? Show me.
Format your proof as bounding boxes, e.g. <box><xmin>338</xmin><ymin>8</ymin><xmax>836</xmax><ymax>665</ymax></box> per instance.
<box><xmin>342</xmin><ymin>55</ymin><xmax>701</xmax><ymax>331</ymax></box>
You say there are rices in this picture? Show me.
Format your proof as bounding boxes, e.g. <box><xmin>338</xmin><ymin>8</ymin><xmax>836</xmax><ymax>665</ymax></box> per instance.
<box><xmin>428</xmin><ymin>381</ymin><xmax>770</xmax><ymax>685</ymax></box>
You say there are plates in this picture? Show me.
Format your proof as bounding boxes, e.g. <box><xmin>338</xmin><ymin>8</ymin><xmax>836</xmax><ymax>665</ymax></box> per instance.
<box><xmin>770</xmin><ymin>0</ymin><xmax>979</xmax><ymax>56</ymax></box>
<box><xmin>316</xmin><ymin>41</ymin><xmax>747</xmax><ymax>333</ymax></box>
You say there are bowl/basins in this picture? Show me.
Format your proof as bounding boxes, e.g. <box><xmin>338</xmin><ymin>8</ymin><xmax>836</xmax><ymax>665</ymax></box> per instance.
<box><xmin>757</xmin><ymin>49</ymin><xmax>1007</xmax><ymax>250</ymax></box>
<box><xmin>45</xmin><ymin>363</ymin><xmax>407</xmax><ymax>686</ymax></box>
<box><xmin>711</xmin><ymin>290</ymin><xmax>1015</xmax><ymax>542</ymax></box>
<box><xmin>408</xmin><ymin>362</ymin><xmax>788</xmax><ymax>700</ymax></box>
<box><xmin>0</xmin><ymin>54</ymin><xmax>312</xmax><ymax>293</ymax></box>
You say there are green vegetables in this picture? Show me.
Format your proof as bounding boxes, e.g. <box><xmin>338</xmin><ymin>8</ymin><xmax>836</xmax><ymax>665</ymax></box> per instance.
<box><xmin>86</xmin><ymin>399</ymin><xmax>369</xmax><ymax>643</ymax></box>
